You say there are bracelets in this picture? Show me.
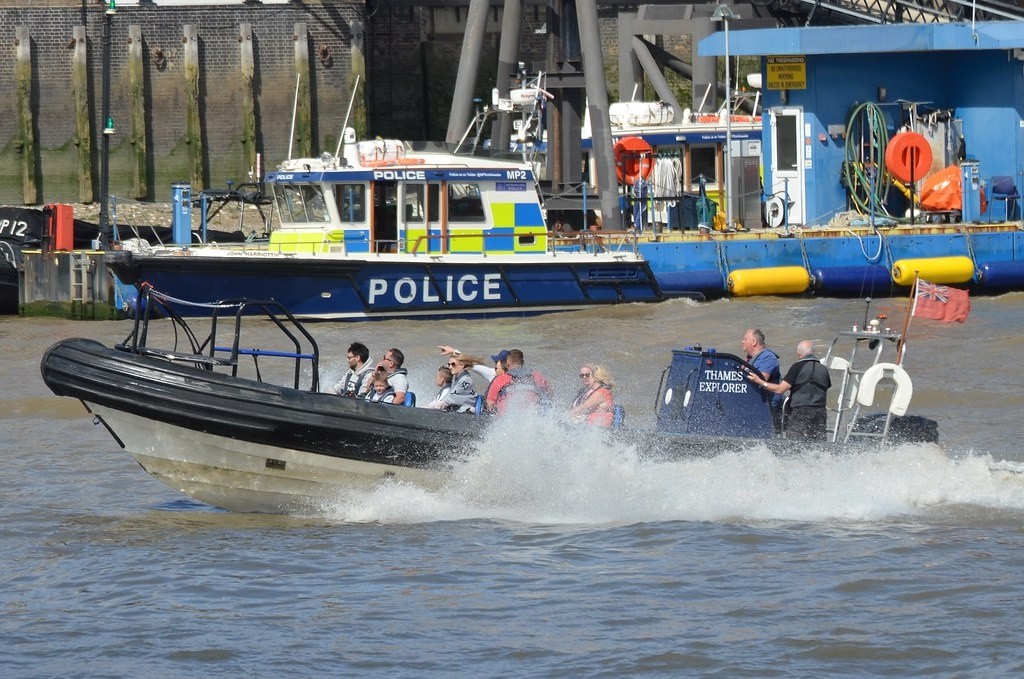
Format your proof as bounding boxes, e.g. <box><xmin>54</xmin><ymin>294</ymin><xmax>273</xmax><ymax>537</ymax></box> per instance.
<box><xmin>763</xmin><ymin>383</ymin><xmax>768</xmax><ymax>389</ymax></box>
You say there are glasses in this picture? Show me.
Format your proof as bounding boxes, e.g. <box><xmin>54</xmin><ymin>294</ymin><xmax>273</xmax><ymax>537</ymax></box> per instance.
<box><xmin>447</xmin><ymin>362</ymin><xmax>460</xmax><ymax>367</ymax></box>
<box><xmin>383</xmin><ymin>355</ymin><xmax>393</xmax><ymax>361</ymax></box>
<box><xmin>347</xmin><ymin>356</ymin><xmax>355</xmax><ymax>360</ymax></box>
<box><xmin>579</xmin><ymin>373</ymin><xmax>591</xmax><ymax>378</ymax></box>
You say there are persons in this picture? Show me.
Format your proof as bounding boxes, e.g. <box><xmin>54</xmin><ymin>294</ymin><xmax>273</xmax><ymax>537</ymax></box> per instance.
<box><xmin>421</xmin><ymin>343</ymin><xmax>554</xmax><ymax>416</ymax></box>
<box><xmin>364</xmin><ymin>372</ymin><xmax>396</xmax><ymax>404</ymax></box>
<box><xmin>366</xmin><ymin>348</ymin><xmax>409</xmax><ymax>405</ymax></box>
<box><xmin>565</xmin><ymin>365</ymin><xmax>616</xmax><ymax>428</ymax></box>
<box><xmin>742</xmin><ymin>327</ymin><xmax>785</xmax><ymax>432</ymax></box>
<box><xmin>746</xmin><ymin>340</ymin><xmax>832</xmax><ymax>442</ymax></box>
<box><xmin>325</xmin><ymin>341</ymin><xmax>376</xmax><ymax>400</ymax></box>
<box><xmin>570</xmin><ymin>362</ymin><xmax>594</xmax><ymax>425</ymax></box>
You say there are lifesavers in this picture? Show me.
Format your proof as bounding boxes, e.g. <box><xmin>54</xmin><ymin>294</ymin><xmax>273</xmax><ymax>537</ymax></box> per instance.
<box><xmin>616</xmin><ymin>151</ymin><xmax>650</xmax><ymax>184</ymax></box>
<box><xmin>856</xmin><ymin>363</ymin><xmax>914</xmax><ymax>416</ymax></box>
<box><xmin>817</xmin><ymin>357</ymin><xmax>860</xmax><ymax>410</ymax></box>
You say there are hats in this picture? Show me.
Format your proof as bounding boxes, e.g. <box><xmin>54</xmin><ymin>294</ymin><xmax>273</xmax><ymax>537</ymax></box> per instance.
<box><xmin>491</xmin><ymin>349</ymin><xmax>511</xmax><ymax>361</ymax></box>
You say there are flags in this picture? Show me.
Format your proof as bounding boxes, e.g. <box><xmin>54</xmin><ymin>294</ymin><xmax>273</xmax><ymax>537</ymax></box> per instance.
<box><xmin>911</xmin><ymin>278</ymin><xmax>970</xmax><ymax>323</ymax></box>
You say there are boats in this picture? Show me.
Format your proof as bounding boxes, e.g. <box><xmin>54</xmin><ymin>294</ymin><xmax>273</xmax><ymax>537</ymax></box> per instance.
<box><xmin>42</xmin><ymin>270</ymin><xmax>969</xmax><ymax>514</ymax></box>
<box><xmin>113</xmin><ymin>20</ymin><xmax>1024</xmax><ymax>313</ymax></box>
<box><xmin>486</xmin><ymin>82</ymin><xmax>765</xmax><ymax>234</ymax></box>
<box><xmin>107</xmin><ymin>73</ymin><xmax>664</xmax><ymax>318</ymax></box>
